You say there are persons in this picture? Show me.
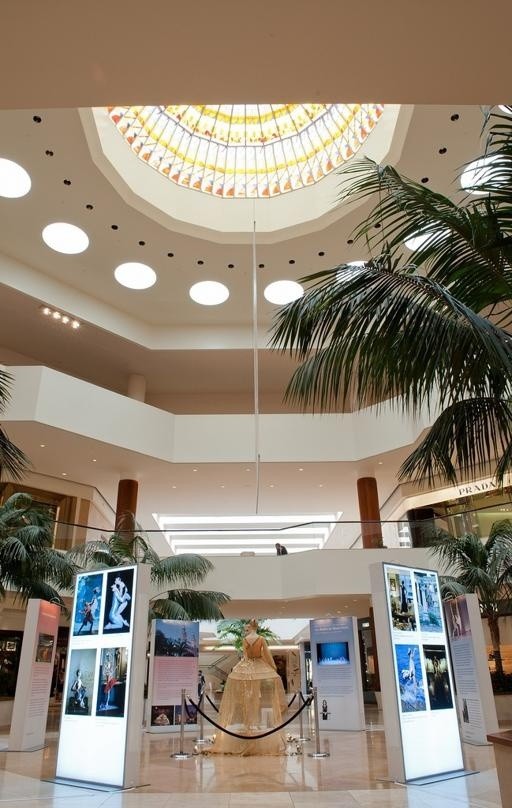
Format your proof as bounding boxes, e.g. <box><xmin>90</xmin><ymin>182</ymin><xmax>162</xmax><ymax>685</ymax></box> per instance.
<box><xmin>399</xmin><ymin>580</ymin><xmax>408</xmax><ymax>612</ymax></box>
<box><xmin>196</xmin><ymin>617</ymin><xmax>290</xmax><ymax>755</ymax></box>
<box><xmin>276</xmin><ymin>543</ymin><xmax>287</xmax><ymax>555</ymax></box>
<box><xmin>322</xmin><ymin>699</ymin><xmax>327</xmax><ymax>720</ymax></box>
<box><xmin>449</xmin><ymin>598</ymin><xmax>465</xmax><ymax>641</ymax></box>
<box><xmin>76</xmin><ymin>577</ymin><xmax>131</xmax><ymax>634</ymax></box>
<box><xmin>198</xmin><ymin>670</ymin><xmax>205</xmax><ymax>697</ymax></box>
<box><xmin>432</xmin><ymin>655</ymin><xmax>442</xmax><ymax>683</ymax></box>
<box><xmin>104</xmin><ymin>660</ymin><xmax>125</xmax><ymax>709</ymax></box>
<box><xmin>71</xmin><ymin>668</ymin><xmax>85</xmax><ymax>709</ymax></box>
<box><xmin>407</xmin><ymin>647</ymin><xmax>418</xmax><ymax>688</ymax></box>
